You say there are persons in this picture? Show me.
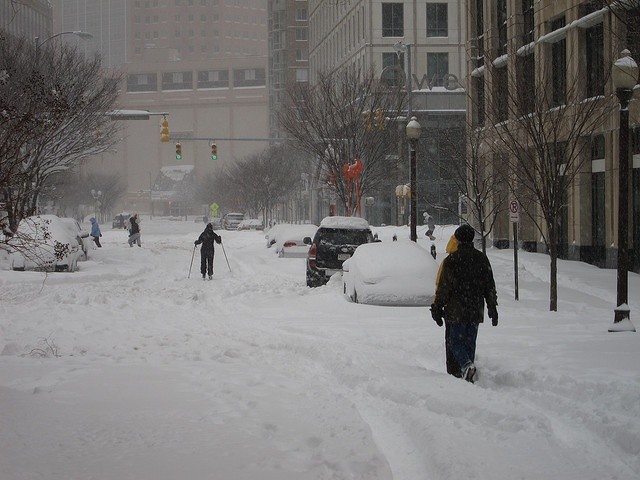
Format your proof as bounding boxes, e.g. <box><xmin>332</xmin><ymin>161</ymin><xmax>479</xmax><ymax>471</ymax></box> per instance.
<box><xmin>90</xmin><ymin>216</ymin><xmax>103</xmax><ymax>248</ymax></box>
<box><xmin>422</xmin><ymin>212</ymin><xmax>436</xmax><ymax>241</ymax></box>
<box><xmin>118</xmin><ymin>214</ymin><xmax>125</xmax><ymax>228</ymax></box>
<box><xmin>431</xmin><ymin>223</ymin><xmax>500</xmax><ymax>384</ymax></box>
<box><xmin>193</xmin><ymin>222</ymin><xmax>222</xmax><ymax>280</ymax></box>
<box><xmin>434</xmin><ymin>236</ymin><xmax>459</xmax><ymax>293</ymax></box>
<box><xmin>128</xmin><ymin>215</ymin><xmax>142</xmax><ymax>247</ymax></box>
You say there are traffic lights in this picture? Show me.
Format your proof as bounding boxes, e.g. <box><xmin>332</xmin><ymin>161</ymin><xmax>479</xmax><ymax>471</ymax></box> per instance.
<box><xmin>211</xmin><ymin>143</ymin><xmax>217</xmax><ymax>160</ymax></box>
<box><xmin>175</xmin><ymin>143</ymin><xmax>182</xmax><ymax>160</ymax></box>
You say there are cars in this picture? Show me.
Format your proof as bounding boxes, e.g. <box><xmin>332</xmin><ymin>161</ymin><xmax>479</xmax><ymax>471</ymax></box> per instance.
<box><xmin>12</xmin><ymin>215</ymin><xmax>74</xmax><ymax>273</ymax></box>
<box><xmin>278</xmin><ymin>241</ymin><xmax>311</xmax><ymax>258</ymax></box>
<box><xmin>264</xmin><ymin>235</ymin><xmax>276</xmax><ymax>249</ymax></box>
<box><xmin>194</xmin><ymin>217</ymin><xmax>204</xmax><ymax>223</ymax></box>
<box><xmin>60</xmin><ymin>218</ymin><xmax>89</xmax><ymax>261</ymax></box>
<box><xmin>250</xmin><ymin>219</ymin><xmax>264</xmax><ymax>230</ymax></box>
<box><xmin>207</xmin><ymin>217</ymin><xmax>223</xmax><ymax>230</ymax></box>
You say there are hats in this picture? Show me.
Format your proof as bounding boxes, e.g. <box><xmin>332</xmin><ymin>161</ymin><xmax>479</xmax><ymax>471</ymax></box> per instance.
<box><xmin>206</xmin><ymin>223</ymin><xmax>212</xmax><ymax>228</ymax></box>
<box><xmin>454</xmin><ymin>225</ymin><xmax>474</xmax><ymax>244</ymax></box>
<box><xmin>423</xmin><ymin>212</ymin><xmax>428</xmax><ymax>216</ymax></box>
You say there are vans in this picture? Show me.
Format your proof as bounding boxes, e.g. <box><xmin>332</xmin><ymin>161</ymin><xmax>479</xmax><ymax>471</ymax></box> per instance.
<box><xmin>112</xmin><ymin>213</ymin><xmax>131</xmax><ymax>229</ymax></box>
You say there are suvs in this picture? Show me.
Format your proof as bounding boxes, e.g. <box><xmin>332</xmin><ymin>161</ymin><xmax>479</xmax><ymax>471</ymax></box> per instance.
<box><xmin>222</xmin><ymin>213</ymin><xmax>244</xmax><ymax>230</ymax></box>
<box><xmin>303</xmin><ymin>225</ymin><xmax>381</xmax><ymax>288</ymax></box>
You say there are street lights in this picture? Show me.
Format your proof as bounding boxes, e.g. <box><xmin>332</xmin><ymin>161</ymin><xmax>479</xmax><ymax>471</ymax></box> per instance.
<box><xmin>91</xmin><ymin>189</ymin><xmax>102</xmax><ymax>224</ymax></box>
<box><xmin>406</xmin><ymin>116</ymin><xmax>422</xmax><ymax>242</ymax></box>
<box><xmin>35</xmin><ymin>29</ymin><xmax>94</xmax><ymax>89</ymax></box>
<box><xmin>606</xmin><ymin>48</ymin><xmax>638</xmax><ymax>332</ymax></box>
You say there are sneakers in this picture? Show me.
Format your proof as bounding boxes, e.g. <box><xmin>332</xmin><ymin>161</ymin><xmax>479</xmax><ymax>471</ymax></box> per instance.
<box><xmin>461</xmin><ymin>363</ymin><xmax>477</xmax><ymax>384</ymax></box>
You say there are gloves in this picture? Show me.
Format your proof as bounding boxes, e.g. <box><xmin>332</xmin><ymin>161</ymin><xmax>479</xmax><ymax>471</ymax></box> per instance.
<box><xmin>432</xmin><ymin>306</ymin><xmax>444</xmax><ymax>327</ymax></box>
<box><xmin>487</xmin><ymin>305</ymin><xmax>498</xmax><ymax>327</ymax></box>
